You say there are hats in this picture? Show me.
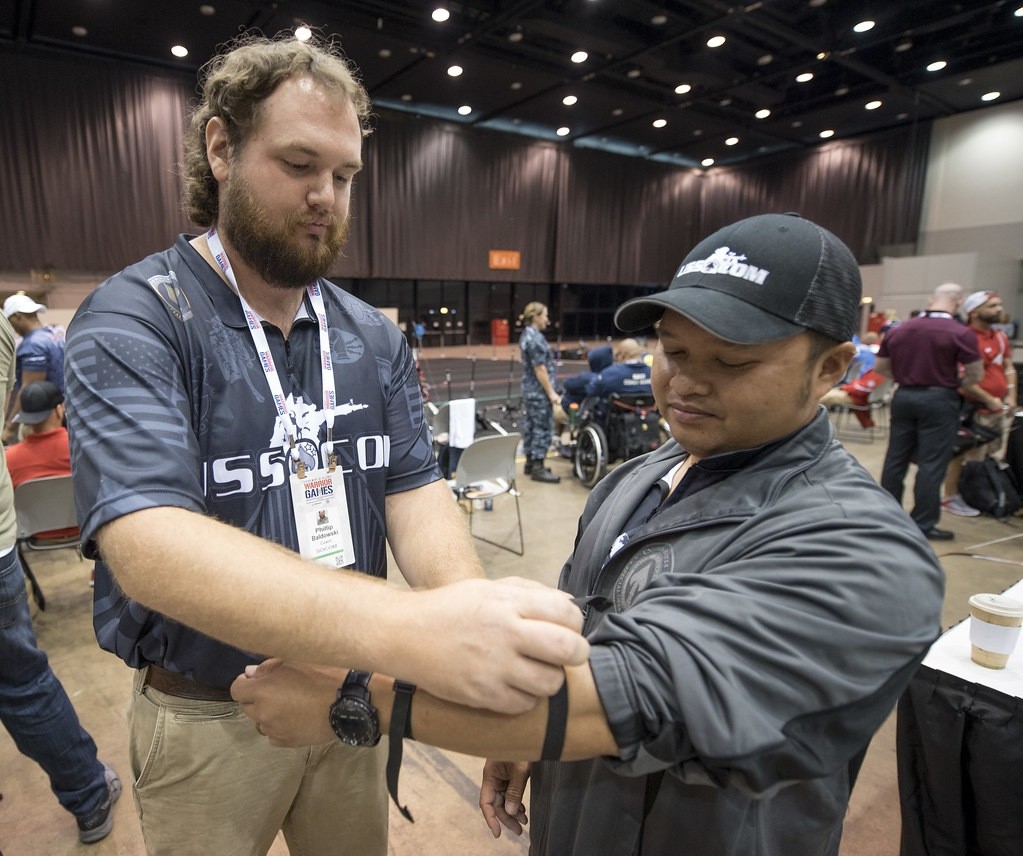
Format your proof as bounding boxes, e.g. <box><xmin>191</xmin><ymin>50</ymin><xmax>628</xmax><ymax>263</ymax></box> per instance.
<box><xmin>614</xmin><ymin>213</ymin><xmax>863</xmax><ymax>345</ymax></box>
<box><xmin>3</xmin><ymin>296</ymin><xmax>47</xmax><ymax>318</ymax></box>
<box><xmin>11</xmin><ymin>380</ymin><xmax>64</xmax><ymax>423</ymax></box>
<box><xmin>965</xmin><ymin>291</ymin><xmax>1000</xmax><ymax>315</ymax></box>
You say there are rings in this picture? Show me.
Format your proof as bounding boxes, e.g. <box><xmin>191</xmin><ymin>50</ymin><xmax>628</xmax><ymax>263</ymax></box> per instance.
<box><xmin>254</xmin><ymin>722</ymin><xmax>265</xmax><ymax>737</ymax></box>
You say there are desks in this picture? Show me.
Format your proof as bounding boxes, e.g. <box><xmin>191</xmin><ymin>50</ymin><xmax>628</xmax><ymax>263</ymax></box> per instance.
<box><xmin>895</xmin><ymin>579</ymin><xmax>1022</xmax><ymax>856</ymax></box>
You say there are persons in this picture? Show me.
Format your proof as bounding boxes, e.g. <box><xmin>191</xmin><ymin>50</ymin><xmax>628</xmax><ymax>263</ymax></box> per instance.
<box><xmin>516</xmin><ymin>301</ymin><xmax>569</xmax><ymax>483</ymax></box>
<box><xmin>875</xmin><ymin>282</ymin><xmax>1017</xmax><ymax>541</ymax></box>
<box><xmin>560</xmin><ymin>337</ymin><xmax>654</xmax><ymax>458</ymax></box>
<box><xmin>64</xmin><ymin>35</ymin><xmax>590</xmax><ymax>856</ymax></box>
<box><xmin>0</xmin><ymin>295</ymin><xmax>121</xmax><ymax>842</ymax></box>
<box><xmin>228</xmin><ymin>211</ymin><xmax>945</xmax><ymax>856</ymax></box>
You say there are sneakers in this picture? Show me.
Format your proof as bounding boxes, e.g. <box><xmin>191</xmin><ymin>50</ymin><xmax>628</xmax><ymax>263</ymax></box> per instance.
<box><xmin>525</xmin><ymin>464</ymin><xmax>550</xmax><ymax>474</ymax></box>
<box><xmin>940</xmin><ymin>494</ymin><xmax>981</xmax><ymax>516</ymax></box>
<box><xmin>530</xmin><ymin>466</ymin><xmax>560</xmax><ymax>482</ymax></box>
<box><xmin>76</xmin><ymin>762</ymin><xmax>122</xmax><ymax>843</ymax></box>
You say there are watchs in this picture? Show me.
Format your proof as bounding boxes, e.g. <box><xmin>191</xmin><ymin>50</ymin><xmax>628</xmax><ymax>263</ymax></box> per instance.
<box><xmin>328</xmin><ymin>669</ymin><xmax>381</xmax><ymax>748</ymax></box>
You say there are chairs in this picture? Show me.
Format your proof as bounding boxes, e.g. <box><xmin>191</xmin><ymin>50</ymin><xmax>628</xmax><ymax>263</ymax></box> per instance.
<box><xmin>445</xmin><ymin>433</ymin><xmax>525</xmax><ymax>558</ymax></box>
<box><xmin>834</xmin><ymin>382</ymin><xmax>901</xmax><ymax>443</ymax></box>
<box><xmin>14</xmin><ymin>474</ymin><xmax>108</xmax><ymax>612</ymax></box>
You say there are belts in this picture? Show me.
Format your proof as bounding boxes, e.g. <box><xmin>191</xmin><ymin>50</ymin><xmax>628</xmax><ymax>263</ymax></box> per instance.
<box><xmin>146</xmin><ymin>662</ymin><xmax>237</xmax><ymax>701</ymax></box>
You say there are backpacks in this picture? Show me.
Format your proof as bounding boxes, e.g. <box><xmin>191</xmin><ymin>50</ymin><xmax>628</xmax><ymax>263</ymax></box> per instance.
<box><xmin>957</xmin><ymin>453</ymin><xmax>1023</xmax><ymax>516</ymax></box>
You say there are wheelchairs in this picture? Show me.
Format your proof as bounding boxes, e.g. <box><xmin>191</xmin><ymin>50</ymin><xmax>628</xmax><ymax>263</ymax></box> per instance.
<box><xmin>557</xmin><ymin>389</ymin><xmax>670</xmax><ymax>490</ymax></box>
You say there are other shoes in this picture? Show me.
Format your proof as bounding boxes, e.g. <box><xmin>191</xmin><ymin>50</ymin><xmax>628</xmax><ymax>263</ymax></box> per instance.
<box><xmin>924</xmin><ymin>528</ymin><xmax>953</xmax><ymax>539</ymax></box>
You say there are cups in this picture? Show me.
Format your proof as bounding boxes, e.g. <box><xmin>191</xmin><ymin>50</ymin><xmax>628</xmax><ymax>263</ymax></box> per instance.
<box><xmin>968</xmin><ymin>593</ymin><xmax>1023</xmax><ymax>670</ymax></box>
